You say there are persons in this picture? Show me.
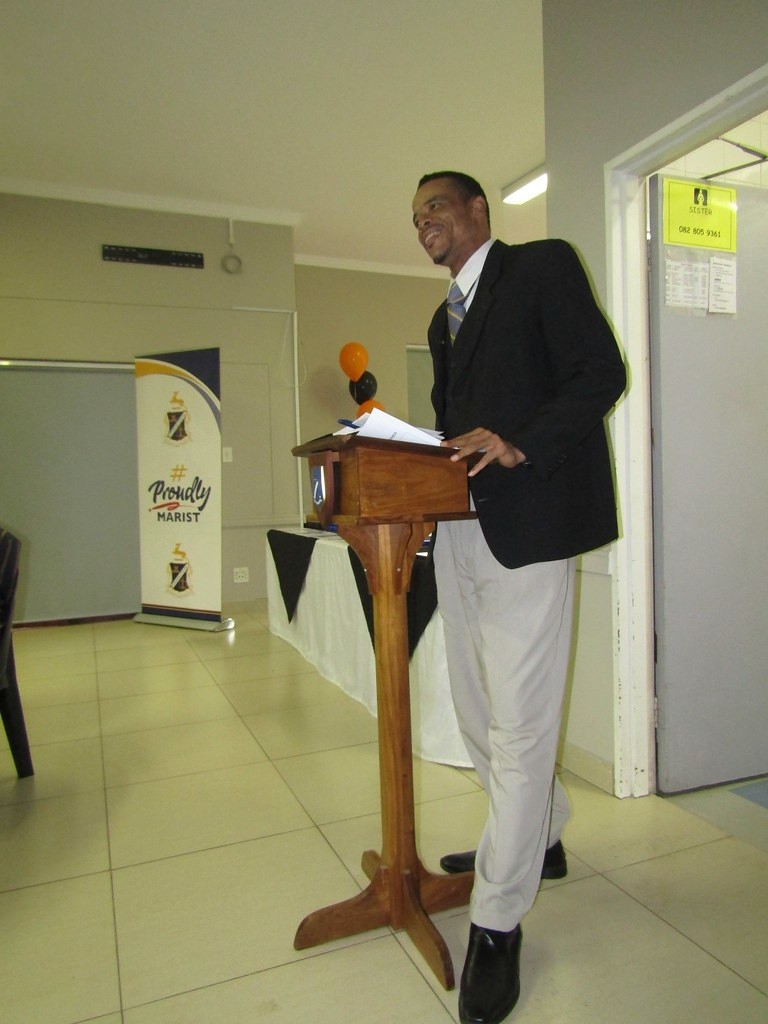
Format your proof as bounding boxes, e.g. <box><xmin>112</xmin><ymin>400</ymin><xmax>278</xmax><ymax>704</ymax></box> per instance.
<box><xmin>412</xmin><ymin>171</ymin><xmax>628</xmax><ymax>1024</ymax></box>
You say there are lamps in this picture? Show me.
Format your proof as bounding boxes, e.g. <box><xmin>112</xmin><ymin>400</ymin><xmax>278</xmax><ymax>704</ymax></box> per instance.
<box><xmin>501</xmin><ymin>163</ymin><xmax>548</xmax><ymax>206</ymax></box>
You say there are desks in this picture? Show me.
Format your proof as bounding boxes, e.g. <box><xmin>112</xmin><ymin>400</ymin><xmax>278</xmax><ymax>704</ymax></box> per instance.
<box><xmin>267</xmin><ymin>527</ymin><xmax>474</xmax><ymax>769</ymax></box>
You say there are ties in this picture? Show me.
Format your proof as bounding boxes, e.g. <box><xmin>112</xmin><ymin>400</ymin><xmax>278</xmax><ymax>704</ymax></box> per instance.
<box><xmin>446</xmin><ymin>281</ymin><xmax>475</xmax><ymax>348</ymax></box>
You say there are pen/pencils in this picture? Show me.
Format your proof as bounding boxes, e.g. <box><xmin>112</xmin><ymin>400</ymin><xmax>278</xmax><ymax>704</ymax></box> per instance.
<box><xmin>338</xmin><ymin>418</ymin><xmax>361</xmax><ymax>429</ymax></box>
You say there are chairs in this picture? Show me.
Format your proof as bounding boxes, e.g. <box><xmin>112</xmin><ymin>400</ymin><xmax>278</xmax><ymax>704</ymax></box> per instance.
<box><xmin>0</xmin><ymin>532</ymin><xmax>34</xmax><ymax>779</ymax></box>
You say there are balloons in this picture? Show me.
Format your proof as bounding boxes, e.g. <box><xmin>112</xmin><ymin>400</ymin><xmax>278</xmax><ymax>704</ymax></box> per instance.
<box><xmin>338</xmin><ymin>341</ymin><xmax>386</xmax><ymax>421</ymax></box>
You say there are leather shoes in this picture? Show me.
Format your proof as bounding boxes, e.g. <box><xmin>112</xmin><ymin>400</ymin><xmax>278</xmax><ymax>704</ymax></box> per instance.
<box><xmin>441</xmin><ymin>840</ymin><xmax>568</xmax><ymax>880</ymax></box>
<box><xmin>459</xmin><ymin>923</ymin><xmax>522</xmax><ymax>1024</ymax></box>
